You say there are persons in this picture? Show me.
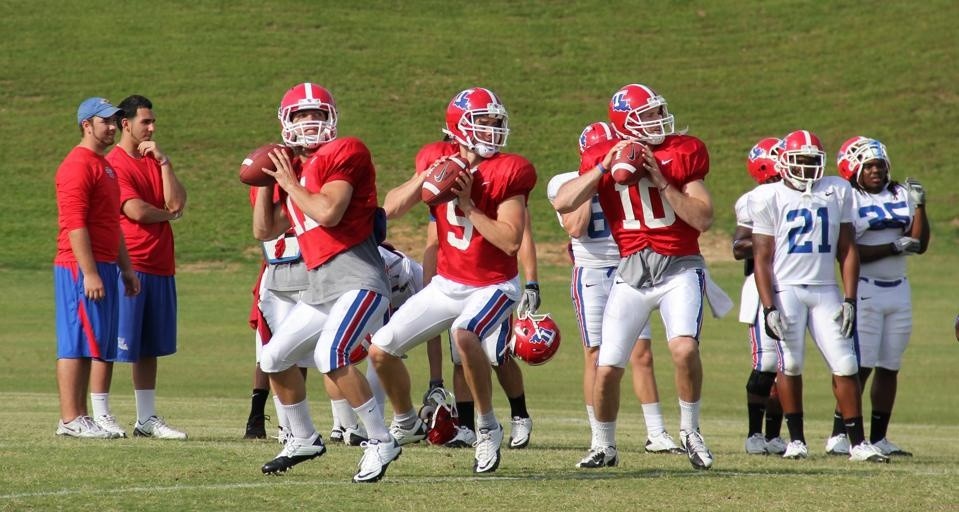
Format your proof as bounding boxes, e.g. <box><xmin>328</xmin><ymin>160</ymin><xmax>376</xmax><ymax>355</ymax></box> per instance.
<box><xmin>51</xmin><ymin>97</ymin><xmax>141</xmax><ymax>439</ymax></box>
<box><xmin>104</xmin><ymin>94</ymin><xmax>190</xmax><ymax>439</ymax></box>
<box><xmin>241</xmin><ymin>80</ymin><xmax>931</xmax><ymax>483</ymax></box>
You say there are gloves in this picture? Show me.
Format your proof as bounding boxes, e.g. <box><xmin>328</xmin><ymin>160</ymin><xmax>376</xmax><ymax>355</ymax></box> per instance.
<box><xmin>834</xmin><ymin>297</ymin><xmax>856</xmax><ymax>338</ymax></box>
<box><xmin>890</xmin><ymin>237</ymin><xmax>921</xmax><ymax>256</ymax></box>
<box><xmin>522</xmin><ymin>282</ymin><xmax>540</xmax><ymax>311</ymax></box>
<box><xmin>763</xmin><ymin>306</ymin><xmax>807</xmax><ymax>337</ymax></box>
<box><xmin>906</xmin><ymin>177</ymin><xmax>926</xmax><ymax>205</ymax></box>
<box><xmin>423</xmin><ymin>383</ymin><xmax>446</xmax><ymax>405</ymax></box>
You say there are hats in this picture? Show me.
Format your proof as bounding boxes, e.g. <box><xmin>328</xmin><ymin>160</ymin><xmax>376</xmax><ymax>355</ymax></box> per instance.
<box><xmin>78</xmin><ymin>97</ymin><xmax>124</xmax><ymax>125</ymax></box>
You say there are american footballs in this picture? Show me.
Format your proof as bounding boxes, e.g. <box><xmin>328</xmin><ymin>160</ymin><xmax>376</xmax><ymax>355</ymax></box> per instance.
<box><xmin>609</xmin><ymin>141</ymin><xmax>648</xmax><ymax>185</ymax></box>
<box><xmin>239</xmin><ymin>144</ymin><xmax>294</xmax><ymax>185</ymax></box>
<box><xmin>422</xmin><ymin>158</ymin><xmax>471</xmax><ymax>204</ymax></box>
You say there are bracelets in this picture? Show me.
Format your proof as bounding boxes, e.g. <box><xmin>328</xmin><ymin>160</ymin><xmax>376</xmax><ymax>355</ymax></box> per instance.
<box><xmin>158</xmin><ymin>157</ymin><xmax>169</xmax><ymax>166</ymax></box>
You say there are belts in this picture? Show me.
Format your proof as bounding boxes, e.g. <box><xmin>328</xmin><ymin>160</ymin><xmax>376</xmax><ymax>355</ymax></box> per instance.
<box><xmin>859</xmin><ymin>277</ymin><xmax>906</xmax><ymax>287</ymax></box>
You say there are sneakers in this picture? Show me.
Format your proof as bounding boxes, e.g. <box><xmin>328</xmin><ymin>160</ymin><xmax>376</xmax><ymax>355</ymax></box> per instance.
<box><xmin>389</xmin><ymin>418</ymin><xmax>427</xmax><ymax>445</ymax></box>
<box><xmin>873</xmin><ymin>437</ymin><xmax>901</xmax><ymax>455</ymax></box>
<box><xmin>330</xmin><ymin>426</ymin><xmax>343</xmax><ymax>442</ymax></box>
<box><xmin>262</xmin><ymin>431</ymin><xmax>326</xmax><ymax>475</ymax></box>
<box><xmin>445</xmin><ymin>424</ymin><xmax>476</xmax><ymax>448</ymax></box>
<box><xmin>783</xmin><ymin>439</ymin><xmax>808</xmax><ymax>458</ymax></box>
<box><xmin>825</xmin><ymin>434</ymin><xmax>849</xmax><ymax>455</ymax></box>
<box><xmin>340</xmin><ymin>424</ymin><xmax>368</xmax><ymax>446</ymax></box>
<box><xmin>278</xmin><ymin>426</ymin><xmax>292</xmax><ymax>443</ymax></box>
<box><xmin>764</xmin><ymin>436</ymin><xmax>786</xmax><ymax>453</ymax></box>
<box><xmin>849</xmin><ymin>441</ymin><xmax>880</xmax><ymax>462</ymax></box>
<box><xmin>472</xmin><ymin>422</ymin><xmax>504</xmax><ymax>473</ymax></box>
<box><xmin>133</xmin><ymin>416</ymin><xmax>188</xmax><ymax>440</ymax></box>
<box><xmin>680</xmin><ymin>425</ymin><xmax>711</xmax><ymax>470</ymax></box>
<box><xmin>645</xmin><ymin>431</ymin><xmax>685</xmax><ymax>454</ymax></box>
<box><xmin>575</xmin><ymin>444</ymin><xmax>618</xmax><ymax>469</ymax></box>
<box><xmin>243</xmin><ymin>415</ymin><xmax>270</xmax><ymax>439</ymax></box>
<box><xmin>55</xmin><ymin>415</ymin><xmax>119</xmax><ymax>438</ymax></box>
<box><xmin>746</xmin><ymin>433</ymin><xmax>764</xmax><ymax>453</ymax></box>
<box><xmin>508</xmin><ymin>416</ymin><xmax>532</xmax><ymax>449</ymax></box>
<box><xmin>94</xmin><ymin>415</ymin><xmax>127</xmax><ymax>438</ymax></box>
<box><xmin>352</xmin><ymin>436</ymin><xmax>402</xmax><ymax>482</ymax></box>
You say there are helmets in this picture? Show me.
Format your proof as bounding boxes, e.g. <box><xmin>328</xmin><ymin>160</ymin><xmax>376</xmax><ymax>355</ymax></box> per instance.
<box><xmin>418</xmin><ymin>405</ymin><xmax>458</xmax><ymax>444</ymax></box>
<box><xmin>578</xmin><ymin>122</ymin><xmax>620</xmax><ymax>157</ymax></box>
<box><xmin>748</xmin><ymin>138</ymin><xmax>783</xmax><ymax>185</ymax></box>
<box><xmin>509</xmin><ymin>311</ymin><xmax>560</xmax><ymax>366</ymax></box>
<box><xmin>837</xmin><ymin>136</ymin><xmax>892</xmax><ymax>191</ymax></box>
<box><xmin>779</xmin><ymin>130</ymin><xmax>826</xmax><ymax>190</ymax></box>
<box><xmin>609</xmin><ymin>84</ymin><xmax>675</xmax><ymax>145</ymax></box>
<box><xmin>441</xmin><ymin>87</ymin><xmax>510</xmax><ymax>158</ymax></box>
<box><xmin>278</xmin><ymin>83</ymin><xmax>339</xmax><ymax>150</ymax></box>
